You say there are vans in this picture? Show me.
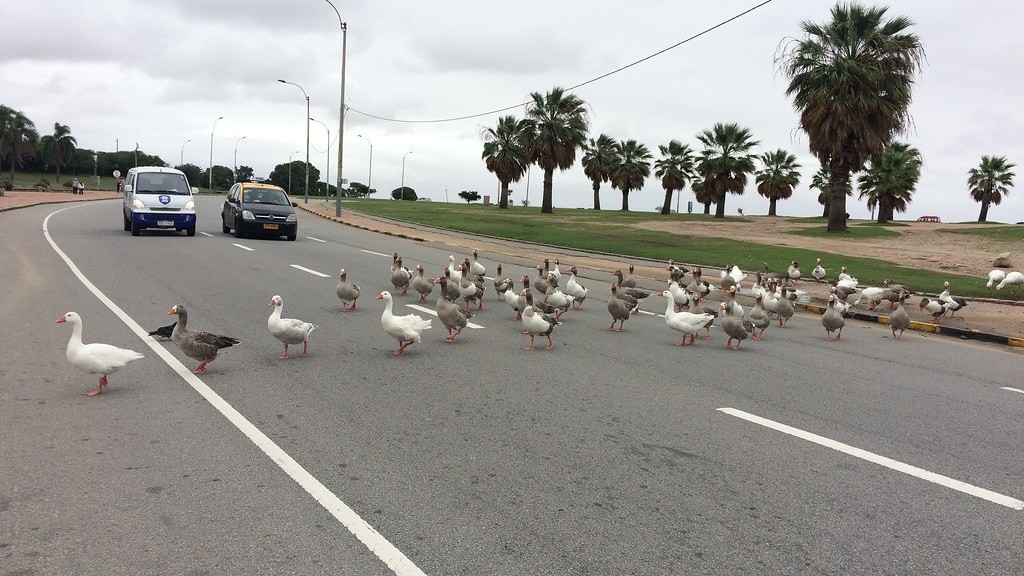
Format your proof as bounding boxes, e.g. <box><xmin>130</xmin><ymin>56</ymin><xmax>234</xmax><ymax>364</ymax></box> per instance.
<box><xmin>916</xmin><ymin>215</ymin><xmax>942</xmax><ymax>222</ymax></box>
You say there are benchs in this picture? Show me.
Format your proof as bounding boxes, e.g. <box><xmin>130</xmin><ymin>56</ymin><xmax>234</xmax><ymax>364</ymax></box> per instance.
<box><xmin>48</xmin><ymin>186</ymin><xmax>70</xmax><ymax>193</ymax></box>
<box><xmin>31</xmin><ymin>186</ymin><xmax>46</xmax><ymax>192</ymax></box>
<box><xmin>13</xmin><ymin>185</ymin><xmax>31</xmax><ymax>192</ymax></box>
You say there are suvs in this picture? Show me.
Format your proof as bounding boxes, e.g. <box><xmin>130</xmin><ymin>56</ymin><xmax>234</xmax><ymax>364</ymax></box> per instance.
<box><xmin>120</xmin><ymin>165</ymin><xmax>200</xmax><ymax>237</ymax></box>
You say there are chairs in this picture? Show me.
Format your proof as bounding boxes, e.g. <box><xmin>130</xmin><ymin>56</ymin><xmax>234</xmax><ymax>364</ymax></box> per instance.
<box><xmin>163</xmin><ymin>177</ymin><xmax>170</xmax><ymax>185</ymax></box>
<box><xmin>138</xmin><ymin>176</ymin><xmax>151</xmax><ymax>185</ymax></box>
<box><xmin>264</xmin><ymin>193</ymin><xmax>280</xmax><ymax>202</ymax></box>
<box><xmin>248</xmin><ymin>192</ymin><xmax>257</xmax><ymax>200</ymax></box>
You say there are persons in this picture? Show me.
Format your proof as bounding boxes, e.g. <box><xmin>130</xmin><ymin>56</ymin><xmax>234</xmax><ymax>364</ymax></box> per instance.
<box><xmin>78</xmin><ymin>181</ymin><xmax>84</xmax><ymax>195</ymax></box>
<box><xmin>72</xmin><ymin>177</ymin><xmax>79</xmax><ymax>195</ymax></box>
<box><xmin>117</xmin><ymin>179</ymin><xmax>121</xmax><ymax>193</ymax></box>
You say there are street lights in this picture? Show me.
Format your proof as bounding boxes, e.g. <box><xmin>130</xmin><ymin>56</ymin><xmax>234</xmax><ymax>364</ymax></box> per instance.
<box><xmin>400</xmin><ymin>151</ymin><xmax>413</xmax><ymax>200</ymax></box>
<box><xmin>288</xmin><ymin>151</ymin><xmax>300</xmax><ymax>195</ymax></box>
<box><xmin>209</xmin><ymin>116</ymin><xmax>224</xmax><ymax>190</ymax></box>
<box><xmin>115</xmin><ymin>138</ymin><xmax>119</xmax><ymax>153</ymax></box>
<box><xmin>358</xmin><ymin>134</ymin><xmax>373</xmax><ymax>198</ymax></box>
<box><xmin>277</xmin><ymin>79</ymin><xmax>309</xmax><ymax>203</ymax></box>
<box><xmin>309</xmin><ymin>118</ymin><xmax>330</xmax><ymax>203</ymax></box>
<box><xmin>180</xmin><ymin>139</ymin><xmax>192</xmax><ymax>166</ymax></box>
<box><xmin>233</xmin><ymin>136</ymin><xmax>247</xmax><ymax>184</ymax></box>
<box><xmin>134</xmin><ymin>141</ymin><xmax>139</xmax><ymax>167</ymax></box>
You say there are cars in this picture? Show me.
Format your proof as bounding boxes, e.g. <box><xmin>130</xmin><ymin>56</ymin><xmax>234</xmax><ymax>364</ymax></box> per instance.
<box><xmin>220</xmin><ymin>181</ymin><xmax>298</xmax><ymax>241</ymax></box>
<box><xmin>416</xmin><ymin>197</ymin><xmax>432</xmax><ymax>202</ymax></box>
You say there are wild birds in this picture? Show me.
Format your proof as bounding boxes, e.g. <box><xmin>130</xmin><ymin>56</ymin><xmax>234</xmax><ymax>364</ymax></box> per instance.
<box><xmin>519</xmin><ymin>288</ymin><xmax>562</xmax><ymax>350</ymax></box>
<box><xmin>55</xmin><ymin>311</ymin><xmax>147</xmax><ymax>398</ymax></box>
<box><xmin>664</xmin><ymin>258</ymin><xmax>827</xmax><ymax>341</ymax></box>
<box><xmin>268</xmin><ymin>294</ymin><xmax>318</xmax><ymax>359</ymax></box>
<box><xmin>336</xmin><ymin>269</ymin><xmax>362</xmax><ymax>311</ymax></box>
<box><xmin>852</xmin><ymin>279</ymin><xmax>916</xmax><ymax>311</ymax></box>
<box><xmin>433</xmin><ymin>276</ymin><xmax>475</xmax><ymax>343</ymax></box>
<box><xmin>147</xmin><ymin>322</ymin><xmax>177</xmax><ymax>341</ymax></box>
<box><xmin>718</xmin><ymin>302</ymin><xmax>756</xmax><ymax>350</ymax></box>
<box><xmin>889</xmin><ymin>291</ymin><xmax>910</xmax><ymax>339</ymax></box>
<box><xmin>390</xmin><ymin>249</ymin><xmax>591</xmax><ymax>322</ymax></box>
<box><xmin>918</xmin><ymin>298</ymin><xmax>953</xmax><ymax>325</ymax></box>
<box><xmin>605</xmin><ymin>264</ymin><xmax>650</xmax><ymax>332</ymax></box>
<box><xmin>166</xmin><ymin>305</ymin><xmax>241</xmax><ymax>374</ymax></box>
<box><xmin>821</xmin><ymin>265</ymin><xmax>860</xmax><ymax>316</ymax></box>
<box><xmin>985</xmin><ymin>269</ymin><xmax>1024</xmax><ymax>292</ymax></box>
<box><xmin>822</xmin><ymin>295</ymin><xmax>846</xmax><ymax>341</ymax></box>
<box><xmin>656</xmin><ymin>291</ymin><xmax>715</xmax><ymax>346</ymax></box>
<box><xmin>940</xmin><ymin>281</ymin><xmax>968</xmax><ymax>318</ymax></box>
<box><xmin>376</xmin><ymin>291</ymin><xmax>433</xmax><ymax>354</ymax></box>
